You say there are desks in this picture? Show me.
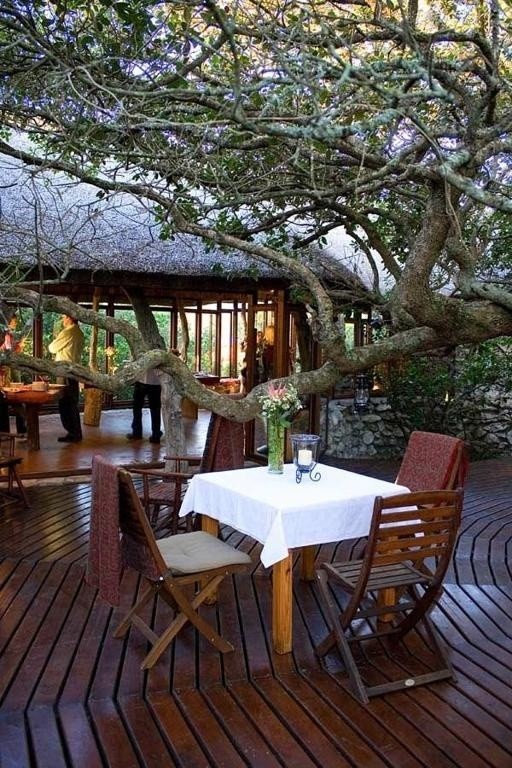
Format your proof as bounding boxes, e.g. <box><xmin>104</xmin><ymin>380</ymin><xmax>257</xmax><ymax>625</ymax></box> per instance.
<box><xmin>191</xmin><ymin>462</ymin><xmax>410</xmax><ymax>657</ymax></box>
<box><xmin>2</xmin><ymin>348</ymin><xmax>253</xmax><ymax>446</ymax></box>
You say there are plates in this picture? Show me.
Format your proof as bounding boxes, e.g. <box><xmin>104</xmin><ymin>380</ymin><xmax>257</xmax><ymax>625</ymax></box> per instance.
<box><xmin>49</xmin><ymin>383</ymin><xmax>67</xmax><ymax>388</ymax></box>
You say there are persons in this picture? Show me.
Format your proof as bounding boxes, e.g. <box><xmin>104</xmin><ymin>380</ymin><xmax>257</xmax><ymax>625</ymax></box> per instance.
<box><xmin>49</xmin><ymin>314</ymin><xmax>85</xmax><ymax>443</ymax></box>
<box><xmin>1</xmin><ymin>314</ymin><xmax>28</xmax><ymax>439</ymax></box>
<box><xmin>127</xmin><ymin>367</ymin><xmax>163</xmax><ymax>444</ymax></box>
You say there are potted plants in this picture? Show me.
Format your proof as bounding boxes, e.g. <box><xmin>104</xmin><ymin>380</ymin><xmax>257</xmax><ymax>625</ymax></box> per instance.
<box><xmin>253</xmin><ymin>379</ymin><xmax>303</xmax><ymax>476</ymax></box>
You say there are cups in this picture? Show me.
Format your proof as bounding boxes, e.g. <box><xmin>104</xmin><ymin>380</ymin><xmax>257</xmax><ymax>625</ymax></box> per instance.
<box><xmin>290</xmin><ymin>433</ymin><xmax>320</xmax><ymax>471</ymax></box>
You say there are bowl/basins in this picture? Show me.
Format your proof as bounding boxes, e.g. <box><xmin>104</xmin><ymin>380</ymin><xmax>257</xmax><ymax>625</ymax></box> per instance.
<box><xmin>3</xmin><ymin>381</ymin><xmax>49</xmax><ymax>392</ymax></box>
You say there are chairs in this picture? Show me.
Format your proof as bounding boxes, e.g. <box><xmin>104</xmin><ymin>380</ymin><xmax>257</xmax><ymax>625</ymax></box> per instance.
<box><xmin>395</xmin><ymin>431</ymin><xmax>465</xmax><ymax>605</ymax></box>
<box><xmin>92</xmin><ymin>456</ymin><xmax>250</xmax><ymax>671</ymax></box>
<box><xmin>132</xmin><ymin>410</ymin><xmax>255</xmax><ymax>545</ymax></box>
<box><xmin>313</xmin><ymin>488</ymin><xmax>464</xmax><ymax>706</ymax></box>
